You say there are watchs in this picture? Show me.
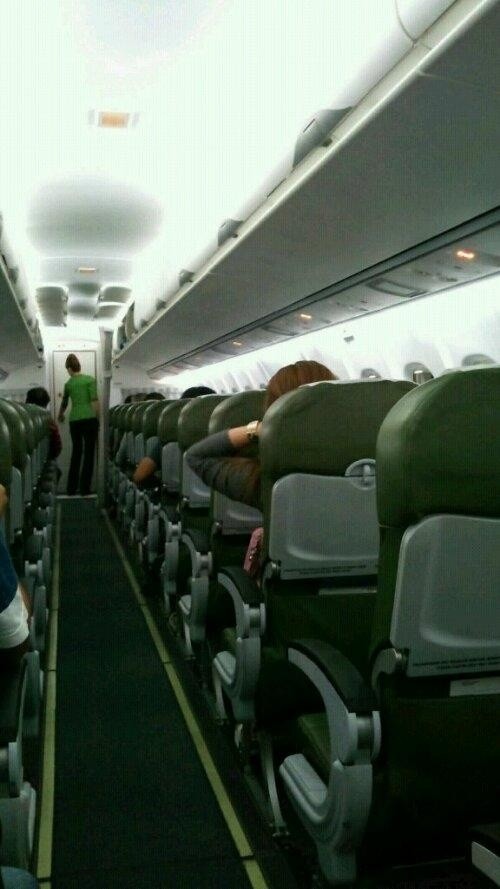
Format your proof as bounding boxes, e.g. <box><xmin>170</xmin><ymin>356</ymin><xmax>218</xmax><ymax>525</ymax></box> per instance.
<box><xmin>245</xmin><ymin>418</ymin><xmax>260</xmax><ymax>442</ymax></box>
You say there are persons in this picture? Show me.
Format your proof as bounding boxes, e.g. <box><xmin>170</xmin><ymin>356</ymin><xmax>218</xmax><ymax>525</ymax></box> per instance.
<box><xmin>26</xmin><ymin>387</ymin><xmax>62</xmax><ymax>459</ymax></box>
<box><xmin>57</xmin><ymin>353</ymin><xmax>102</xmax><ymax>497</ymax></box>
<box><xmin>108</xmin><ymin>385</ymin><xmax>216</xmax><ymax>498</ymax></box>
<box><xmin>1</xmin><ymin>484</ymin><xmax>33</xmax><ymax>673</ymax></box>
<box><xmin>185</xmin><ymin>357</ymin><xmax>334</xmax><ymax>596</ymax></box>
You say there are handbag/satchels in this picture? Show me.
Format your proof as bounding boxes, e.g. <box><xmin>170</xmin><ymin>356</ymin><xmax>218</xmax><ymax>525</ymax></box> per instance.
<box><xmin>244</xmin><ymin>525</ymin><xmax>265</xmax><ymax>573</ymax></box>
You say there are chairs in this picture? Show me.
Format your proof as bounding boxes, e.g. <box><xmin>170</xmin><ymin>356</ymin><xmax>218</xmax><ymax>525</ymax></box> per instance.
<box><xmin>0</xmin><ymin>396</ymin><xmax>55</xmax><ymax>889</ymax></box>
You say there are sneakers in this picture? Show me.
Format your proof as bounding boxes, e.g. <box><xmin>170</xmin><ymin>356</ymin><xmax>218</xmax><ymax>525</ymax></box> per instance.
<box><xmin>67</xmin><ymin>486</ymin><xmax>93</xmax><ymax>495</ymax></box>
